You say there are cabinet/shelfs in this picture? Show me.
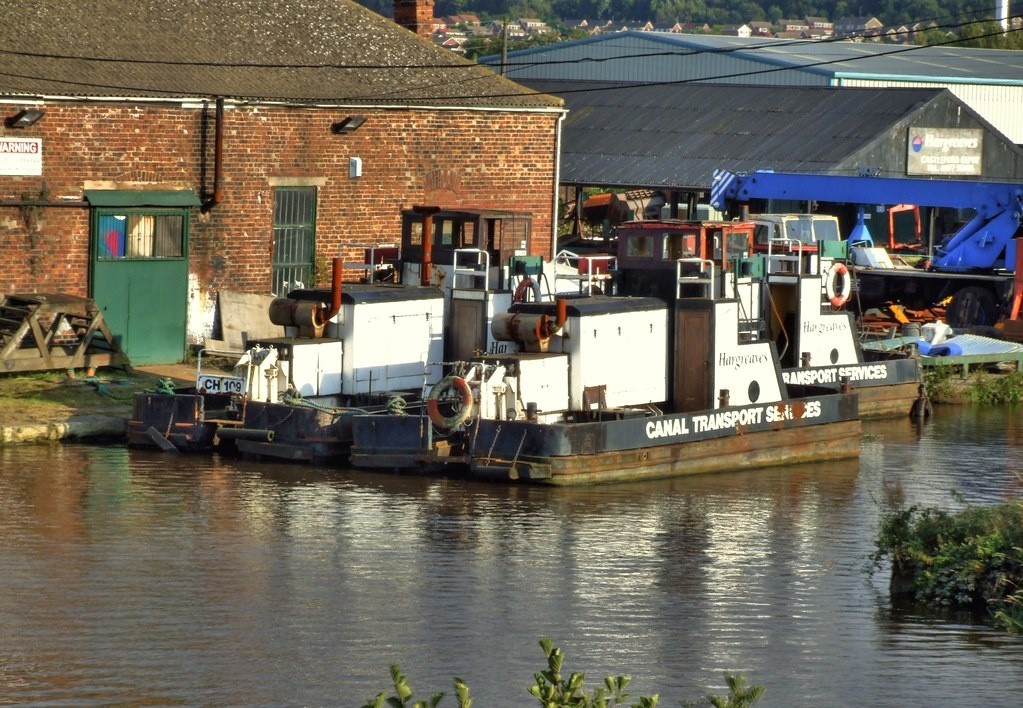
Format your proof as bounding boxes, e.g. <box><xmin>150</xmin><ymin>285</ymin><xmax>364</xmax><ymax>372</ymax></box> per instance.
<box><xmin>245</xmin><ymin>336</ymin><xmax>344</xmax><ymax>398</ymax></box>
<box><xmin>471</xmin><ymin>351</ymin><xmax>571</xmax><ymax>415</ymax></box>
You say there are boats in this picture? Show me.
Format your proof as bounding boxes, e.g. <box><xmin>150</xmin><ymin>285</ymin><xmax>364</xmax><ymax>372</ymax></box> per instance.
<box><xmin>121</xmin><ymin>189</ymin><xmax>1023</xmax><ymax>489</ymax></box>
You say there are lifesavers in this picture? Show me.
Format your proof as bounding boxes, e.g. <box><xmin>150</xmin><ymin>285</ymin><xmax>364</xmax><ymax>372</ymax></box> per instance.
<box><xmin>514</xmin><ymin>277</ymin><xmax>542</xmax><ymax>302</ymax></box>
<box><xmin>824</xmin><ymin>262</ymin><xmax>851</xmax><ymax>307</ymax></box>
<box><xmin>913</xmin><ymin>398</ymin><xmax>935</xmax><ymax>418</ymax></box>
<box><xmin>426</xmin><ymin>375</ymin><xmax>474</xmax><ymax>429</ymax></box>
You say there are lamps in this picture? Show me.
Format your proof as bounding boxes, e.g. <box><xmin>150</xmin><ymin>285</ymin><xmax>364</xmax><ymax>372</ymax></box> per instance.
<box><xmin>331</xmin><ymin>116</ymin><xmax>368</xmax><ymax>133</ymax></box>
<box><xmin>4</xmin><ymin>109</ymin><xmax>45</xmax><ymax>129</ymax></box>
